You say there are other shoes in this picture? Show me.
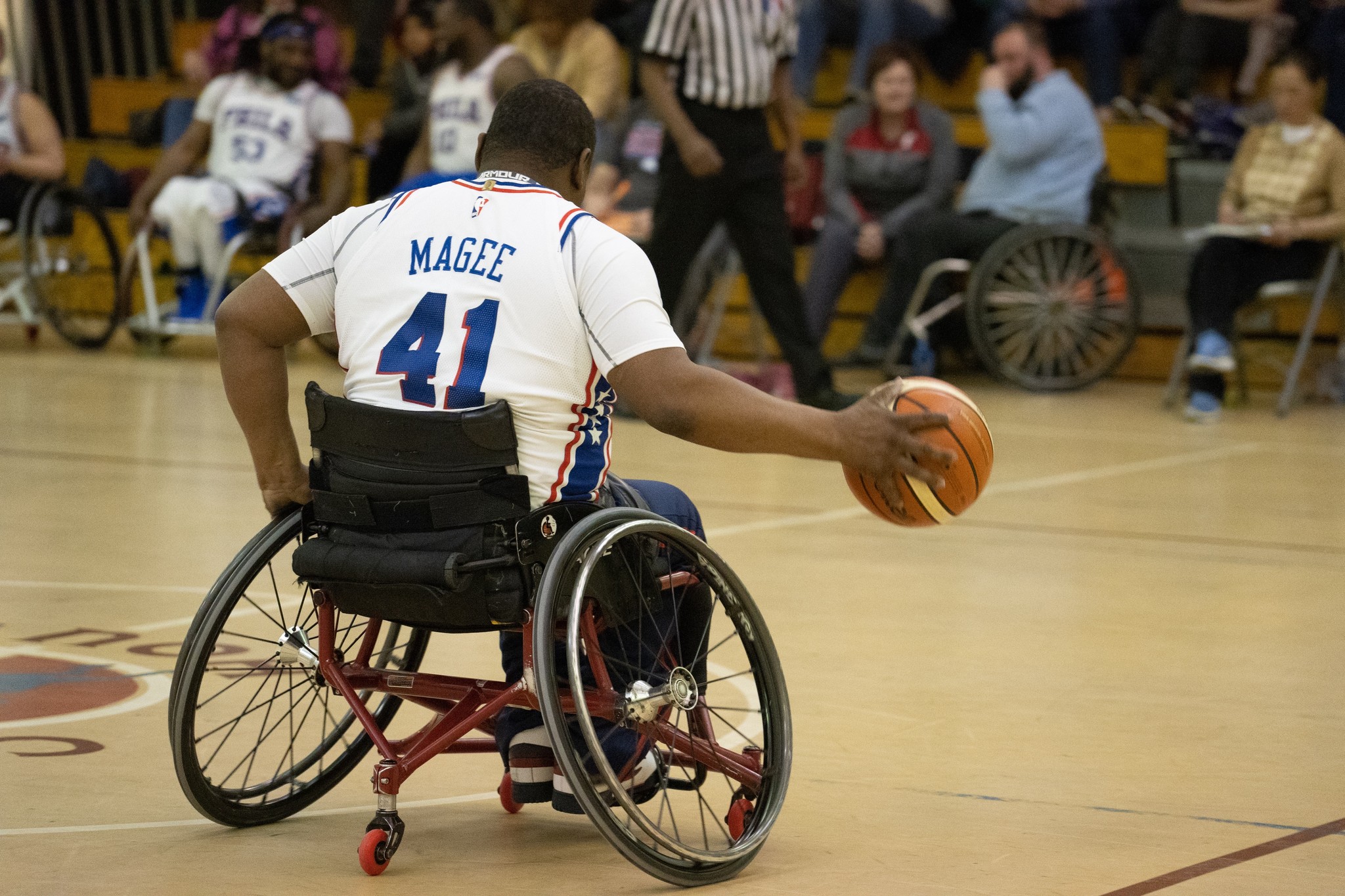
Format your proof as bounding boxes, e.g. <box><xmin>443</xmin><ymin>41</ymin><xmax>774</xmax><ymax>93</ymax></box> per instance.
<box><xmin>1185</xmin><ymin>390</ymin><xmax>1220</xmax><ymax>421</ymax></box>
<box><xmin>553</xmin><ymin>745</ymin><xmax>665</xmax><ymax>813</ymax></box>
<box><xmin>799</xmin><ymin>384</ymin><xmax>863</xmax><ymax>412</ymax></box>
<box><xmin>508</xmin><ymin>725</ymin><xmax>553</xmax><ymax>803</ymax></box>
<box><xmin>168</xmin><ymin>274</ymin><xmax>233</xmax><ymax>325</ymax></box>
<box><xmin>1189</xmin><ymin>331</ymin><xmax>1237</xmax><ymax>373</ymax></box>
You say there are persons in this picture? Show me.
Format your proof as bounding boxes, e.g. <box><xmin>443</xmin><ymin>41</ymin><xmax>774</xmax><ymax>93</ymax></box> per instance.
<box><xmin>1</xmin><ymin>0</ymin><xmax>1345</xmax><ymax>423</ymax></box>
<box><xmin>211</xmin><ymin>78</ymin><xmax>962</xmax><ymax>812</ymax></box>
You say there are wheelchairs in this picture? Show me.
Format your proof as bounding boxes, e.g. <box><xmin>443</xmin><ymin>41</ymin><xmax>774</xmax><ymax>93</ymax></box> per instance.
<box><xmin>166</xmin><ymin>377</ymin><xmax>797</xmax><ymax>887</ymax></box>
<box><xmin>876</xmin><ymin>152</ymin><xmax>1144</xmax><ymax>392</ymax></box>
<box><xmin>118</xmin><ymin>172</ymin><xmax>326</xmax><ymax>350</ymax></box>
<box><xmin>0</xmin><ymin>168</ymin><xmax>126</xmax><ymax>352</ymax></box>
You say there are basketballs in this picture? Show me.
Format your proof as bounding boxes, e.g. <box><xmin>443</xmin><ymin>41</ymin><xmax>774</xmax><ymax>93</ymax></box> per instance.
<box><xmin>842</xmin><ymin>375</ymin><xmax>994</xmax><ymax>526</ymax></box>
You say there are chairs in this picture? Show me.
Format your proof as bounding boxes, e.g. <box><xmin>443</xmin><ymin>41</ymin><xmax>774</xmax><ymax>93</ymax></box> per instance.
<box><xmin>1160</xmin><ymin>238</ymin><xmax>1345</xmax><ymax>417</ymax></box>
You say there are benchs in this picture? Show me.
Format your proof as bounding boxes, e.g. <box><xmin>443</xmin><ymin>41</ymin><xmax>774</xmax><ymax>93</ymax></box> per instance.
<box><xmin>0</xmin><ymin>0</ymin><xmax>1173</xmax><ymax>364</ymax></box>
